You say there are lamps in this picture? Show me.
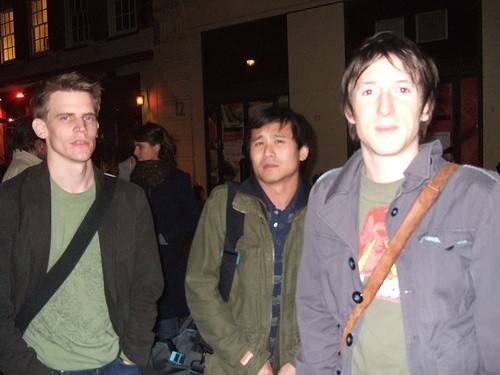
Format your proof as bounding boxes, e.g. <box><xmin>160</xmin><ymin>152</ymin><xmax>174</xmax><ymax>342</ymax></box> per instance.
<box><xmin>136</xmin><ymin>89</ymin><xmax>149</xmax><ymax>107</ymax></box>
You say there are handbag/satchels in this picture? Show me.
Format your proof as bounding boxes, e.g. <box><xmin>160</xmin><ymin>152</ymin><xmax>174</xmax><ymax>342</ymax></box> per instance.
<box><xmin>149</xmin><ymin>179</ymin><xmax>246</xmax><ymax>375</ymax></box>
